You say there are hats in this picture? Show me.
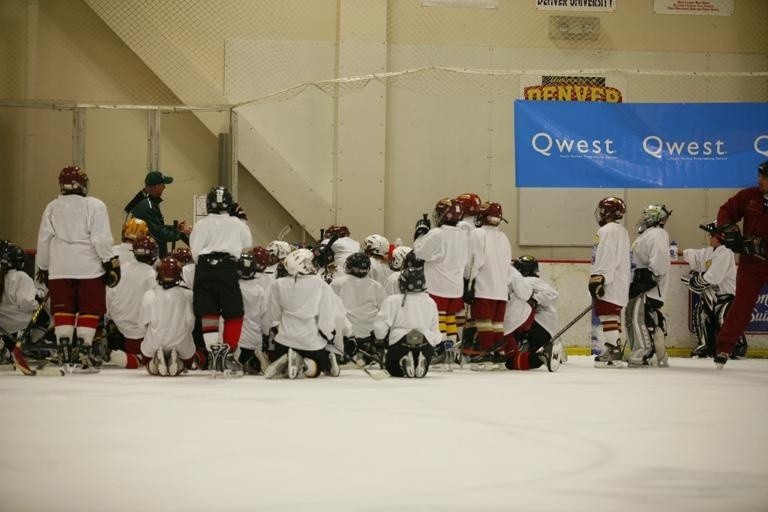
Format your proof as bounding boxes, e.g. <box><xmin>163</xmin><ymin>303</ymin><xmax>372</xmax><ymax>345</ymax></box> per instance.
<box><xmin>145</xmin><ymin>171</ymin><xmax>173</xmax><ymax>186</ymax></box>
<box><xmin>758</xmin><ymin>160</ymin><xmax>767</xmax><ymax>177</ymax></box>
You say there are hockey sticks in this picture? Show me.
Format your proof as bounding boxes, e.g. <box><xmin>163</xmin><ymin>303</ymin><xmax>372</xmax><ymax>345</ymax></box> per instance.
<box><xmin>11</xmin><ymin>290</ymin><xmax>50</xmax><ymax>375</ymax></box>
<box><xmin>331</xmin><ymin>343</ymin><xmax>391</xmax><ymax>380</ymax></box>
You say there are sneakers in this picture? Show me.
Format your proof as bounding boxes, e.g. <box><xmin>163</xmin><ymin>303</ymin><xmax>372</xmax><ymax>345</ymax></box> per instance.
<box><xmin>74</xmin><ymin>338</ymin><xmax>102</xmax><ymax>368</ymax></box>
<box><xmin>714</xmin><ymin>355</ymin><xmax>727</xmax><ymax>364</ymax></box>
<box><xmin>595</xmin><ymin>353</ymin><xmax>622</xmax><ymax>361</ymax></box>
<box><xmin>430</xmin><ymin>352</ymin><xmax>445</xmax><ymax>364</ymax></box>
<box><xmin>56</xmin><ymin>336</ymin><xmax>71</xmax><ymax>363</ymax></box>
<box><xmin>222</xmin><ymin>345</ymin><xmax>243</xmax><ymax>370</ymax></box>
<box><xmin>691</xmin><ymin>345</ymin><xmax>715</xmax><ymax>358</ymax></box>
<box><xmin>169</xmin><ymin>350</ymin><xmax>179</xmax><ymax>376</ymax></box>
<box><xmin>156</xmin><ymin>348</ymin><xmax>168</xmax><ymax>376</ymax></box>
<box><xmin>471</xmin><ymin>353</ymin><xmax>506</xmax><ymax>363</ymax></box>
<box><xmin>264</xmin><ymin>354</ymin><xmax>288</xmax><ymax>379</ymax></box>
<box><xmin>208</xmin><ymin>343</ymin><xmax>221</xmax><ymax>371</ymax></box>
<box><xmin>416</xmin><ymin>355</ymin><xmax>427</xmax><ymax>378</ymax></box>
<box><xmin>402</xmin><ymin>354</ymin><xmax>415</xmax><ymax>377</ymax></box>
<box><xmin>627</xmin><ymin>354</ymin><xmax>646</xmax><ymax>364</ymax></box>
<box><xmin>289</xmin><ymin>348</ymin><xmax>305</xmax><ymax>379</ymax></box>
<box><xmin>254</xmin><ymin>350</ymin><xmax>270</xmax><ymax>370</ymax></box>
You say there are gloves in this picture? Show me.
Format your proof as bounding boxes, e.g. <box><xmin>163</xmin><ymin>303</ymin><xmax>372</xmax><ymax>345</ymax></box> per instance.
<box><xmin>103</xmin><ymin>256</ymin><xmax>121</xmax><ymax>288</ymax></box>
<box><xmin>414</xmin><ymin>219</ymin><xmax>430</xmax><ymax>240</ymax></box>
<box><xmin>233</xmin><ymin>202</ymin><xmax>246</xmax><ymax>219</ymax></box>
<box><xmin>742</xmin><ymin>235</ymin><xmax>765</xmax><ymax>257</ymax></box>
<box><xmin>589</xmin><ymin>275</ymin><xmax>605</xmax><ymax>300</ymax></box>
<box><xmin>722</xmin><ymin>224</ymin><xmax>741</xmax><ymax>251</ymax></box>
<box><xmin>36</xmin><ymin>269</ymin><xmax>48</xmax><ymax>285</ymax></box>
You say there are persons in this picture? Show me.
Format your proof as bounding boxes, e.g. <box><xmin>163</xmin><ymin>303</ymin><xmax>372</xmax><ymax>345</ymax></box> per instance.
<box><xmin>102</xmin><ymin>173</ymin><xmax>558</xmax><ymax>376</ymax></box>
<box><xmin>677</xmin><ymin>221</ymin><xmax>747</xmax><ymax>358</ymax></box>
<box><xmin>716</xmin><ymin>161</ymin><xmax>768</xmax><ymax>364</ymax></box>
<box><xmin>626</xmin><ymin>204</ymin><xmax>671</xmax><ymax>369</ymax></box>
<box><xmin>584</xmin><ymin>196</ymin><xmax>632</xmax><ymax>368</ymax></box>
<box><xmin>0</xmin><ymin>240</ymin><xmax>54</xmax><ymax>376</ymax></box>
<box><xmin>35</xmin><ymin>166</ymin><xmax>120</xmax><ymax>368</ymax></box>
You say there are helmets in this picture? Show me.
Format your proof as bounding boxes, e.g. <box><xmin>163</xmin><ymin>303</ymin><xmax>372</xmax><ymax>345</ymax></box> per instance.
<box><xmin>133</xmin><ymin>235</ymin><xmax>159</xmax><ymax>261</ymax></box>
<box><xmin>433</xmin><ymin>197</ymin><xmax>461</xmax><ymax>226</ymax></box>
<box><xmin>344</xmin><ymin>252</ymin><xmax>371</xmax><ymax>278</ymax></box>
<box><xmin>157</xmin><ymin>256</ymin><xmax>182</xmax><ymax>283</ymax></box>
<box><xmin>59</xmin><ymin>165</ymin><xmax>88</xmax><ymax>196</ymax></box>
<box><xmin>458</xmin><ymin>192</ymin><xmax>481</xmax><ymax>218</ymax></box>
<box><xmin>363</xmin><ymin>234</ymin><xmax>390</xmax><ymax>258</ymax></box>
<box><xmin>402</xmin><ymin>249</ymin><xmax>424</xmax><ymax>269</ymax></box>
<box><xmin>477</xmin><ymin>201</ymin><xmax>503</xmax><ymax>226</ymax></box>
<box><xmin>172</xmin><ymin>247</ymin><xmax>191</xmax><ymax>264</ymax></box>
<box><xmin>325</xmin><ymin>224</ymin><xmax>350</xmax><ymax>238</ymax></box>
<box><xmin>283</xmin><ymin>248</ymin><xmax>316</xmax><ymax>276</ymax></box>
<box><xmin>7</xmin><ymin>245</ymin><xmax>26</xmax><ymax>270</ymax></box>
<box><xmin>253</xmin><ymin>246</ymin><xmax>270</xmax><ymax>272</ymax></box>
<box><xmin>398</xmin><ymin>267</ymin><xmax>426</xmax><ymax>293</ymax></box>
<box><xmin>237</xmin><ymin>254</ymin><xmax>256</xmax><ymax>278</ymax></box>
<box><xmin>637</xmin><ymin>201</ymin><xmax>672</xmax><ymax>233</ymax></box>
<box><xmin>514</xmin><ymin>255</ymin><xmax>538</xmax><ymax>276</ymax></box>
<box><xmin>206</xmin><ymin>187</ymin><xmax>232</xmax><ymax>214</ymax></box>
<box><xmin>391</xmin><ymin>246</ymin><xmax>415</xmax><ymax>269</ymax></box>
<box><xmin>594</xmin><ymin>196</ymin><xmax>626</xmax><ymax>227</ymax></box>
<box><xmin>267</xmin><ymin>240</ymin><xmax>291</xmax><ymax>260</ymax></box>
<box><xmin>123</xmin><ymin>219</ymin><xmax>149</xmax><ymax>241</ymax></box>
